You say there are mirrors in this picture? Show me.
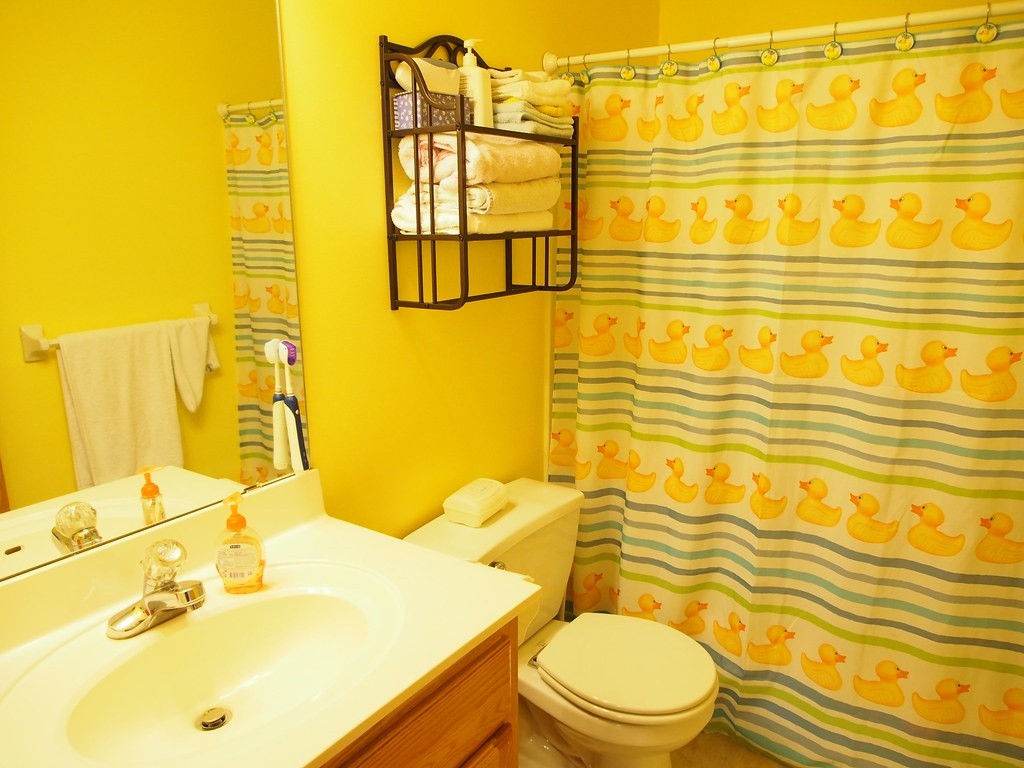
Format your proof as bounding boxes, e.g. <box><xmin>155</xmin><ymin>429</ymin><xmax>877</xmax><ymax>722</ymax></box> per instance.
<box><xmin>0</xmin><ymin>1</ymin><xmax>312</xmax><ymax>585</ymax></box>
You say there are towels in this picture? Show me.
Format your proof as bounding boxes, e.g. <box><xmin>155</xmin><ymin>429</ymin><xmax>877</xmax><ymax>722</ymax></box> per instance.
<box><xmin>58</xmin><ymin>319</ymin><xmax>183</xmax><ymax>488</ymax></box>
<box><xmin>169</xmin><ymin>303</ymin><xmax>219</xmax><ymax>413</ymax></box>
<box><xmin>390</xmin><ymin>55</ymin><xmax>573</xmax><ymax>240</ymax></box>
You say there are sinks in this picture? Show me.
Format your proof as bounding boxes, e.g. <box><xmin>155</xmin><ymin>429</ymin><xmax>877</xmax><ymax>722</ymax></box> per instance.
<box><xmin>1</xmin><ymin>560</ymin><xmax>407</xmax><ymax>768</ymax></box>
<box><xmin>0</xmin><ymin>467</ymin><xmax>250</xmax><ymax>578</ymax></box>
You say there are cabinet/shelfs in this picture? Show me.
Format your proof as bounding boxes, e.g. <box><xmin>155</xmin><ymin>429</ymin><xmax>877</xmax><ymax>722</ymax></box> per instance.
<box><xmin>314</xmin><ymin>616</ymin><xmax>522</xmax><ymax>768</ymax></box>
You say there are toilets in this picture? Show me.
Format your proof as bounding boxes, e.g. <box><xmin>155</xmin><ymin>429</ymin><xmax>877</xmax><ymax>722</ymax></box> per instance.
<box><xmin>400</xmin><ymin>472</ymin><xmax>721</xmax><ymax>768</ymax></box>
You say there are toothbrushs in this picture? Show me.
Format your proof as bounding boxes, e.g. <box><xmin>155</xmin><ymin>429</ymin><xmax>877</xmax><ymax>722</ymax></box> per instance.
<box><xmin>265</xmin><ymin>339</ymin><xmax>290</xmax><ymax>471</ymax></box>
<box><xmin>279</xmin><ymin>341</ymin><xmax>309</xmax><ymax>472</ymax></box>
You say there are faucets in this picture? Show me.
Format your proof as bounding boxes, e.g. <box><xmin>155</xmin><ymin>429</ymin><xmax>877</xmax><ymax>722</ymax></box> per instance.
<box><xmin>106</xmin><ymin>538</ymin><xmax>207</xmax><ymax>638</ymax></box>
<box><xmin>50</xmin><ymin>499</ymin><xmax>104</xmax><ymax>554</ymax></box>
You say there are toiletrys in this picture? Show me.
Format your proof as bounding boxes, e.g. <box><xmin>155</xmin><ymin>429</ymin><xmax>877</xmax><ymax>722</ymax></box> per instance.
<box><xmin>216</xmin><ymin>490</ymin><xmax>264</xmax><ymax>592</ymax></box>
<box><xmin>140</xmin><ymin>466</ymin><xmax>168</xmax><ymax>526</ymax></box>
